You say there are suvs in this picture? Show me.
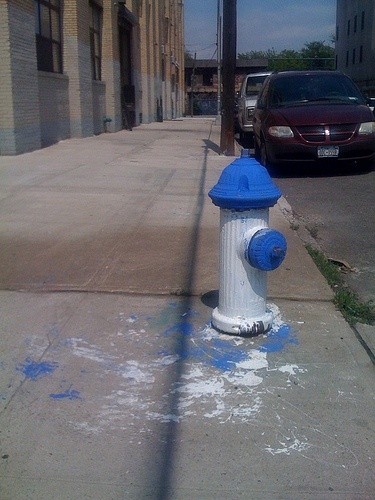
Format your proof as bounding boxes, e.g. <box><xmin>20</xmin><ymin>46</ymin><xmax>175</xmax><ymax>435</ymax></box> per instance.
<box><xmin>236</xmin><ymin>71</ymin><xmax>276</xmax><ymax>146</ymax></box>
<box><xmin>252</xmin><ymin>67</ymin><xmax>375</xmax><ymax>176</ymax></box>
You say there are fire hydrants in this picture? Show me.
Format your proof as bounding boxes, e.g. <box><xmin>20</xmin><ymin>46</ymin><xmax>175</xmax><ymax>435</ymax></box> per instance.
<box><xmin>209</xmin><ymin>147</ymin><xmax>286</xmax><ymax>337</ymax></box>
